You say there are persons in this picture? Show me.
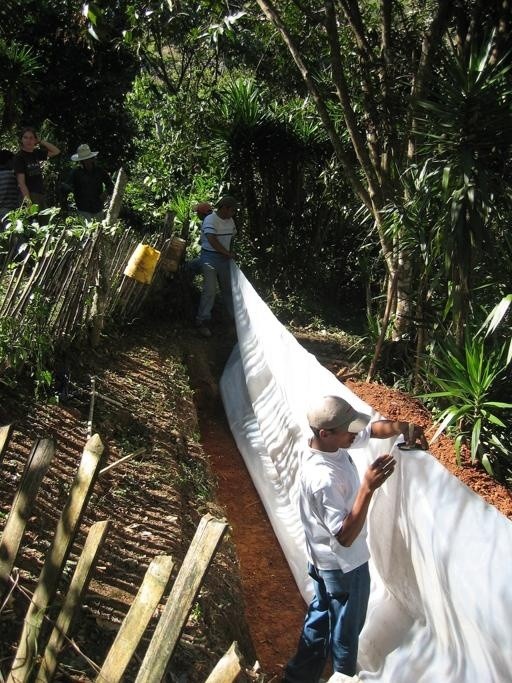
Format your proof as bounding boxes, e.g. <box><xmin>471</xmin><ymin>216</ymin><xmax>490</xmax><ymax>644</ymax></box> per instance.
<box><xmin>166</xmin><ymin>201</ymin><xmax>215</xmax><ymax>296</ymax></box>
<box><xmin>283</xmin><ymin>394</ymin><xmax>430</xmax><ymax>683</ymax></box>
<box><xmin>195</xmin><ymin>195</ymin><xmax>238</xmax><ymax>338</ymax></box>
<box><xmin>0</xmin><ymin>147</ymin><xmax>21</xmax><ymax>231</ymax></box>
<box><xmin>13</xmin><ymin>125</ymin><xmax>62</xmax><ymax>206</ymax></box>
<box><xmin>61</xmin><ymin>143</ymin><xmax>116</xmax><ymax>222</ymax></box>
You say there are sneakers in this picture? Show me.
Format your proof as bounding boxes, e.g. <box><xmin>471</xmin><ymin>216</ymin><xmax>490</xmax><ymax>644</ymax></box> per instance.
<box><xmin>195</xmin><ymin>319</ymin><xmax>211</xmax><ymax>337</ymax></box>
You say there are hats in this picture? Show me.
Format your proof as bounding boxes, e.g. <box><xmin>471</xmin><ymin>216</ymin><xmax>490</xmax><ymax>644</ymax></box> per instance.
<box><xmin>192</xmin><ymin>201</ymin><xmax>213</xmax><ymax>214</ymax></box>
<box><xmin>220</xmin><ymin>194</ymin><xmax>241</xmax><ymax>207</ymax></box>
<box><xmin>306</xmin><ymin>394</ymin><xmax>371</xmax><ymax>433</ymax></box>
<box><xmin>71</xmin><ymin>143</ymin><xmax>100</xmax><ymax>161</ymax></box>
